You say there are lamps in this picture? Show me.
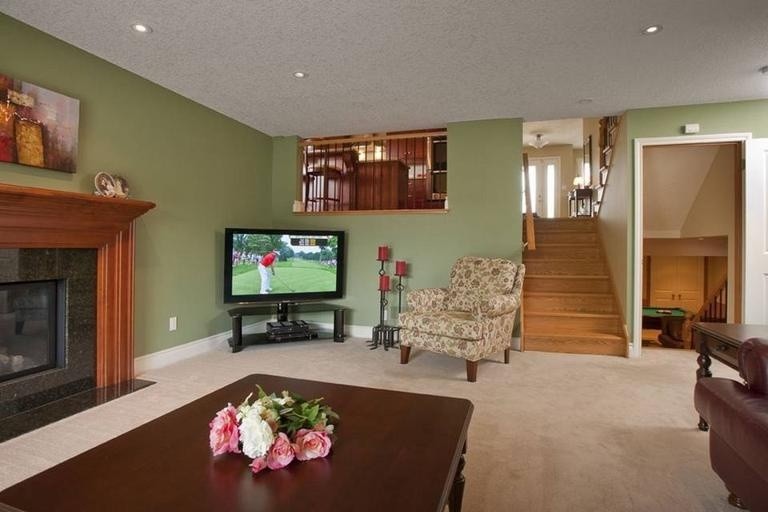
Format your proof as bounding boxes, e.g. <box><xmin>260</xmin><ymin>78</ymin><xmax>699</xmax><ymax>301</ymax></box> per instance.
<box><xmin>527</xmin><ymin>132</ymin><xmax>549</xmax><ymax>149</ymax></box>
<box><xmin>571</xmin><ymin>174</ymin><xmax>583</xmax><ymax>189</ymax></box>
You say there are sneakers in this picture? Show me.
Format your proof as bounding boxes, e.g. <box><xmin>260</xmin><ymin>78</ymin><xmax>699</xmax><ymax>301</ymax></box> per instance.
<box><xmin>260</xmin><ymin>287</ymin><xmax>273</xmax><ymax>295</ymax></box>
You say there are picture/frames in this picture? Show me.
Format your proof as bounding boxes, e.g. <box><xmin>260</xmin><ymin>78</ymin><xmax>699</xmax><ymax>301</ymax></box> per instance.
<box><xmin>582</xmin><ymin>135</ymin><xmax>593</xmax><ymax>188</ymax></box>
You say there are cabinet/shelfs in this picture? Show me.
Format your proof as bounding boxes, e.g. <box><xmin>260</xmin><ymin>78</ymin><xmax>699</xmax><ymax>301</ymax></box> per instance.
<box><xmin>227</xmin><ymin>303</ymin><xmax>348</xmax><ymax>353</ymax></box>
<box><xmin>386</xmin><ymin>130</ymin><xmax>426</xmax><ymax>166</ymax></box>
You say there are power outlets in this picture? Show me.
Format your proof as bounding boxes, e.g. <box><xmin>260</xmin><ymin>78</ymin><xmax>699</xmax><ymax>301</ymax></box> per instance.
<box><xmin>167</xmin><ymin>315</ymin><xmax>178</xmax><ymax>332</ymax></box>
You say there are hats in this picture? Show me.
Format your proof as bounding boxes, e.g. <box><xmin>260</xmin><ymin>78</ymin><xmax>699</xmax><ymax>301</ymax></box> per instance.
<box><xmin>273</xmin><ymin>249</ymin><xmax>281</xmax><ymax>256</ymax></box>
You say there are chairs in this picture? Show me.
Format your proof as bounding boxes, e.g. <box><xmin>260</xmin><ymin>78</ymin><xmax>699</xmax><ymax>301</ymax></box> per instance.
<box><xmin>694</xmin><ymin>331</ymin><xmax>768</xmax><ymax>512</ymax></box>
<box><xmin>396</xmin><ymin>254</ymin><xmax>526</xmax><ymax>384</ymax></box>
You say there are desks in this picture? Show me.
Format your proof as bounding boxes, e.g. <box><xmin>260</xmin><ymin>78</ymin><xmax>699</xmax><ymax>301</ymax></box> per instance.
<box><xmin>567</xmin><ymin>188</ymin><xmax>593</xmax><ymax>218</ymax></box>
<box><xmin>688</xmin><ymin>318</ymin><xmax>768</xmax><ymax>432</ymax></box>
<box><xmin>642</xmin><ymin>307</ymin><xmax>690</xmax><ymax>348</ymax></box>
<box><xmin>0</xmin><ymin>369</ymin><xmax>474</xmax><ymax>510</ymax></box>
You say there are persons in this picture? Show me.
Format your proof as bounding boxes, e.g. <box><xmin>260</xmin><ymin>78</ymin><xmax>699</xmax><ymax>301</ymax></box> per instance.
<box><xmin>257</xmin><ymin>249</ymin><xmax>281</xmax><ymax>296</ymax></box>
<box><xmin>233</xmin><ymin>249</ymin><xmax>262</xmax><ymax>264</ymax></box>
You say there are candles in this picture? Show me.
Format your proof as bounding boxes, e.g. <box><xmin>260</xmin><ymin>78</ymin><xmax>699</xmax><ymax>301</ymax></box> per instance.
<box><xmin>375</xmin><ymin>245</ymin><xmax>407</xmax><ymax>292</ymax></box>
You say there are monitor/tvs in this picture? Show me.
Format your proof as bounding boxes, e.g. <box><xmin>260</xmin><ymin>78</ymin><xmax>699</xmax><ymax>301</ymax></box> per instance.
<box><xmin>223</xmin><ymin>228</ymin><xmax>346</xmax><ymax>305</ymax></box>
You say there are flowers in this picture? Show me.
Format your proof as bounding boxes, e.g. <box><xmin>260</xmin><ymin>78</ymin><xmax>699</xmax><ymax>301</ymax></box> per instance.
<box><xmin>203</xmin><ymin>383</ymin><xmax>338</xmax><ymax>477</ymax></box>
